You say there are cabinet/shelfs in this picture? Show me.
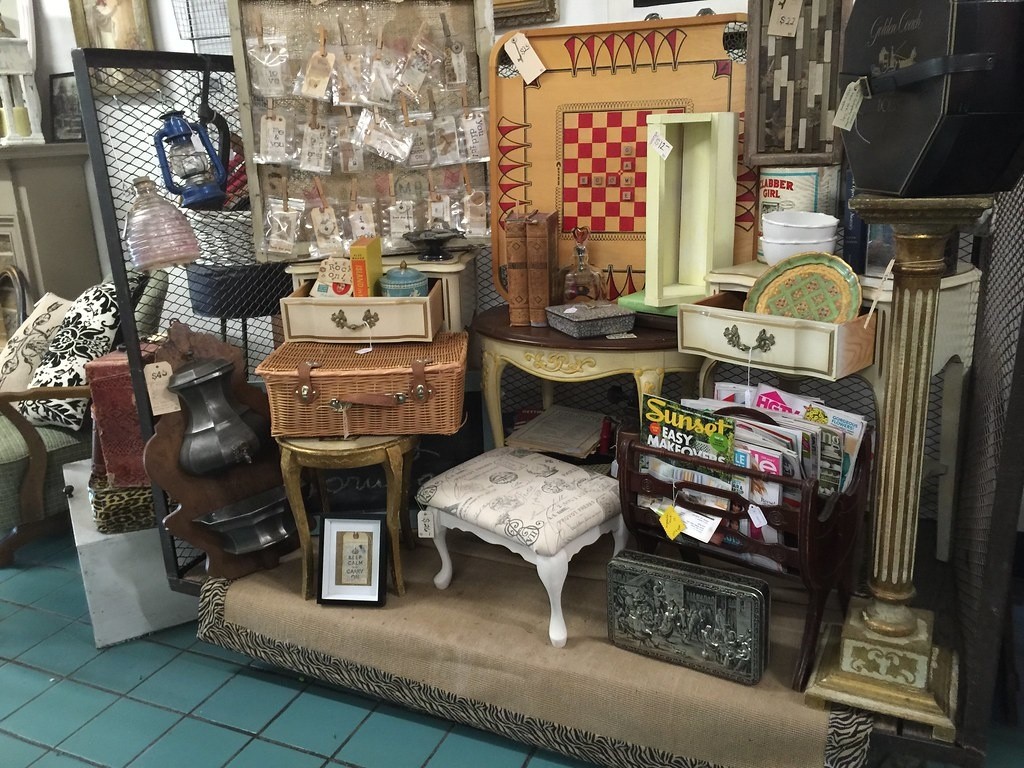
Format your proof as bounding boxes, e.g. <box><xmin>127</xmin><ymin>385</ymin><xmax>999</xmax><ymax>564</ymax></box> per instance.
<box><xmin>279</xmin><ymin>247</ymin><xmax>481</xmax><ymax>344</ymax></box>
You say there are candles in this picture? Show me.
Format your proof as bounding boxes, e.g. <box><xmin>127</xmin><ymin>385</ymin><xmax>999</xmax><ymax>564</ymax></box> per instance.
<box><xmin>0</xmin><ymin>107</ymin><xmax>32</xmax><ymax>137</ymax></box>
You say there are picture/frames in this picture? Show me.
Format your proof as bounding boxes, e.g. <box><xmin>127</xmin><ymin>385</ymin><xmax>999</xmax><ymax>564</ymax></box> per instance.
<box><xmin>68</xmin><ymin>0</ymin><xmax>160</xmax><ymax>97</ymax></box>
<box><xmin>49</xmin><ymin>72</ymin><xmax>85</xmax><ymax>143</ymax></box>
<box><xmin>316</xmin><ymin>512</ymin><xmax>387</xmax><ymax>608</ymax></box>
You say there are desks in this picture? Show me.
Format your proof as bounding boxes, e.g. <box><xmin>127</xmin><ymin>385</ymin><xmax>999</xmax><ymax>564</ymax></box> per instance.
<box><xmin>276</xmin><ymin>434</ymin><xmax>416</xmax><ymax>601</ymax></box>
<box><xmin>471</xmin><ymin>304</ymin><xmax>702</xmax><ymax>447</ymax></box>
<box><xmin>679</xmin><ymin>257</ymin><xmax>981</xmax><ymax>562</ymax></box>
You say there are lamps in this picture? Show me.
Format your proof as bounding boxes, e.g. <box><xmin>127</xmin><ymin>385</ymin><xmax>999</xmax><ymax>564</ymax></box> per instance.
<box><xmin>153</xmin><ymin>108</ymin><xmax>231</xmax><ymax>208</ymax></box>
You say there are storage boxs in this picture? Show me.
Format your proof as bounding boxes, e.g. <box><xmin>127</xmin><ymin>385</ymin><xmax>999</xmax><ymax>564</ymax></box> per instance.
<box><xmin>607</xmin><ymin>550</ymin><xmax>770</xmax><ymax>687</ymax></box>
<box><xmin>645</xmin><ymin>110</ymin><xmax>740</xmax><ymax>307</ymax></box>
<box><xmin>742</xmin><ymin>0</ymin><xmax>854</xmax><ymax>166</ymax></box>
<box><xmin>61</xmin><ymin>458</ymin><xmax>198</xmax><ymax>649</ymax></box>
<box><xmin>83</xmin><ymin>339</ymin><xmax>167</xmax><ymax>487</ymax></box>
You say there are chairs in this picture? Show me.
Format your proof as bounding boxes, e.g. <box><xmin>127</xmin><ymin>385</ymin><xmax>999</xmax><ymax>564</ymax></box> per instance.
<box><xmin>0</xmin><ymin>267</ymin><xmax>170</xmax><ymax>569</ymax></box>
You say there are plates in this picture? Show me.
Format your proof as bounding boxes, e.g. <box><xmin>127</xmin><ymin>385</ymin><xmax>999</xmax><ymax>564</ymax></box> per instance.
<box><xmin>742</xmin><ymin>253</ymin><xmax>862</xmax><ymax>324</ymax></box>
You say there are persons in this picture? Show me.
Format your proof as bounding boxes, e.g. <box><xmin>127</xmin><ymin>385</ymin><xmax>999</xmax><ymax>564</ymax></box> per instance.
<box><xmin>729</xmin><ymin>519</ymin><xmax>740</xmax><ymax>531</ymax></box>
<box><xmin>737</xmin><ymin>443</ymin><xmax>764</xmax><ymax>542</ymax></box>
<box><xmin>731</xmin><ymin>501</ymin><xmax>743</xmax><ymax>513</ymax></box>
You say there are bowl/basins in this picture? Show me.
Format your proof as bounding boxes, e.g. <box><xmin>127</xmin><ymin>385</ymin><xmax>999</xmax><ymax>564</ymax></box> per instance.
<box><xmin>761</xmin><ymin>210</ymin><xmax>840</xmax><ymax>241</ymax></box>
<box><xmin>760</xmin><ymin>237</ymin><xmax>837</xmax><ymax>267</ymax></box>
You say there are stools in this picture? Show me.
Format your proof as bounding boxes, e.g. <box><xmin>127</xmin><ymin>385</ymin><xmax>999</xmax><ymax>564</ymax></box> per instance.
<box><xmin>417</xmin><ymin>446</ymin><xmax>624</xmax><ymax>648</ymax></box>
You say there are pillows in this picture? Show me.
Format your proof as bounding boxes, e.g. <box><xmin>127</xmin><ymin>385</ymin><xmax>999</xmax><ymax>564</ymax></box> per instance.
<box><xmin>15</xmin><ymin>268</ymin><xmax>146</xmax><ymax>435</ymax></box>
<box><xmin>0</xmin><ymin>290</ymin><xmax>73</xmax><ymax>415</ymax></box>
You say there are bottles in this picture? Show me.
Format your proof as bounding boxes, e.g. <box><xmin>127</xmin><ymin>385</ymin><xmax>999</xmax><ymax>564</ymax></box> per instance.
<box><xmin>125</xmin><ymin>177</ymin><xmax>200</xmax><ymax>272</ymax></box>
<box><xmin>557</xmin><ymin>227</ymin><xmax>607</xmax><ymax>304</ymax></box>
<box><xmin>380</xmin><ymin>260</ymin><xmax>428</xmax><ymax>298</ymax></box>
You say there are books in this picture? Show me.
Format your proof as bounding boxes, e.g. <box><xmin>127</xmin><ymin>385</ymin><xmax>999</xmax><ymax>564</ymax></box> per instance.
<box><xmin>505</xmin><ymin>406</ymin><xmax>619</xmax><ymax>459</ymax></box>
<box><xmin>638</xmin><ymin>381</ymin><xmax>868</xmax><ymax>570</ymax></box>
